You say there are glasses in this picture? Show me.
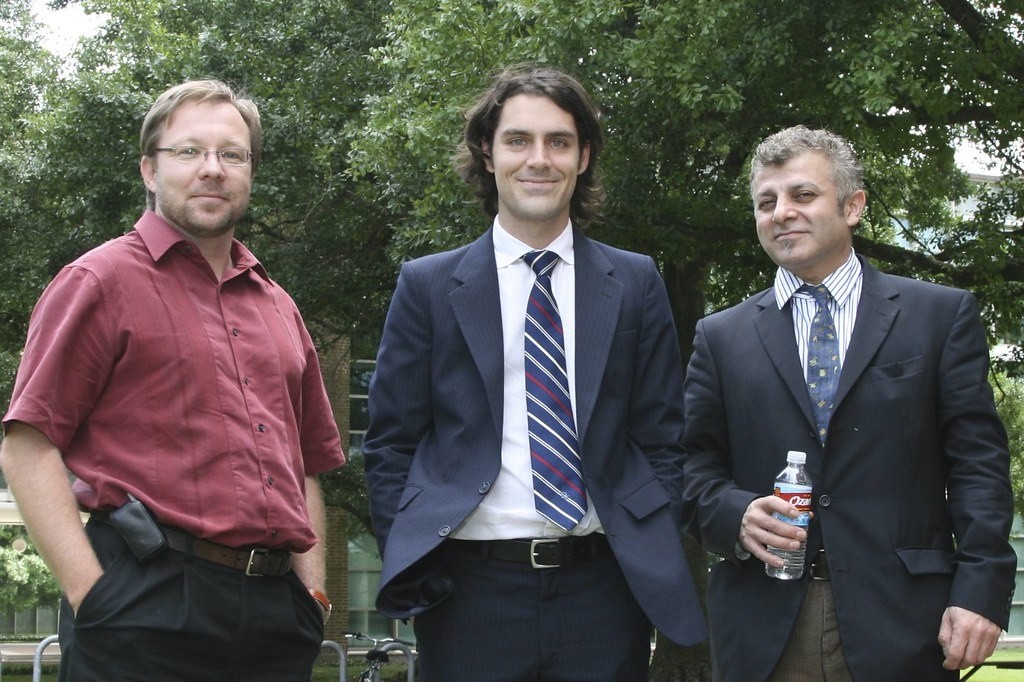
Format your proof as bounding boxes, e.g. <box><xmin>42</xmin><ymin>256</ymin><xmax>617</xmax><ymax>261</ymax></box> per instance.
<box><xmin>152</xmin><ymin>145</ymin><xmax>253</xmax><ymax>167</ymax></box>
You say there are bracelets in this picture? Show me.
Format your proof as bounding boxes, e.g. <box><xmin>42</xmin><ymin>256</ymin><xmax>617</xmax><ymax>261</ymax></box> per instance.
<box><xmin>304</xmin><ymin>588</ymin><xmax>332</xmax><ymax>626</ymax></box>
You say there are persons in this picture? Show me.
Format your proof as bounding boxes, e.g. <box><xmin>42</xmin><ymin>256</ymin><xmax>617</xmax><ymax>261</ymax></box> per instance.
<box><xmin>361</xmin><ymin>67</ymin><xmax>687</xmax><ymax>681</ymax></box>
<box><xmin>0</xmin><ymin>77</ymin><xmax>347</xmax><ymax>682</ymax></box>
<box><xmin>683</xmin><ymin>125</ymin><xmax>1019</xmax><ymax>682</ymax></box>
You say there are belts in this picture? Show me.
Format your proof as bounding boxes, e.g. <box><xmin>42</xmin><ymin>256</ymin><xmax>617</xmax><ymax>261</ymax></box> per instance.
<box><xmin>441</xmin><ymin>536</ymin><xmax>611</xmax><ymax>569</ymax></box>
<box><xmin>90</xmin><ymin>507</ymin><xmax>292</xmax><ymax>576</ymax></box>
<box><xmin>808</xmin><ymin>549</ymin><xmax>831</xmax><ymax>580</ymax></box>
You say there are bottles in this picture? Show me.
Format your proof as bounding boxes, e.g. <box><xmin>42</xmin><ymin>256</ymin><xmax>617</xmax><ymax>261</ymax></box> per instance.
<box><xmin>765</xmin><ymin>451</ymin><xmax>813</xmax><ymax>580</ymax></box>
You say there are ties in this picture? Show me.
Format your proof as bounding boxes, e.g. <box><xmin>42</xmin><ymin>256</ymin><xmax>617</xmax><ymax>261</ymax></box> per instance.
<box><xmin>798</xmin><ymin>284</ymin><xmax>841</xmax><ymax>448</ymax></box>
<box><xmin>518</xmin><ymin>251</ymin><xmax>589</xmax><ymax>532</ymax></box>
